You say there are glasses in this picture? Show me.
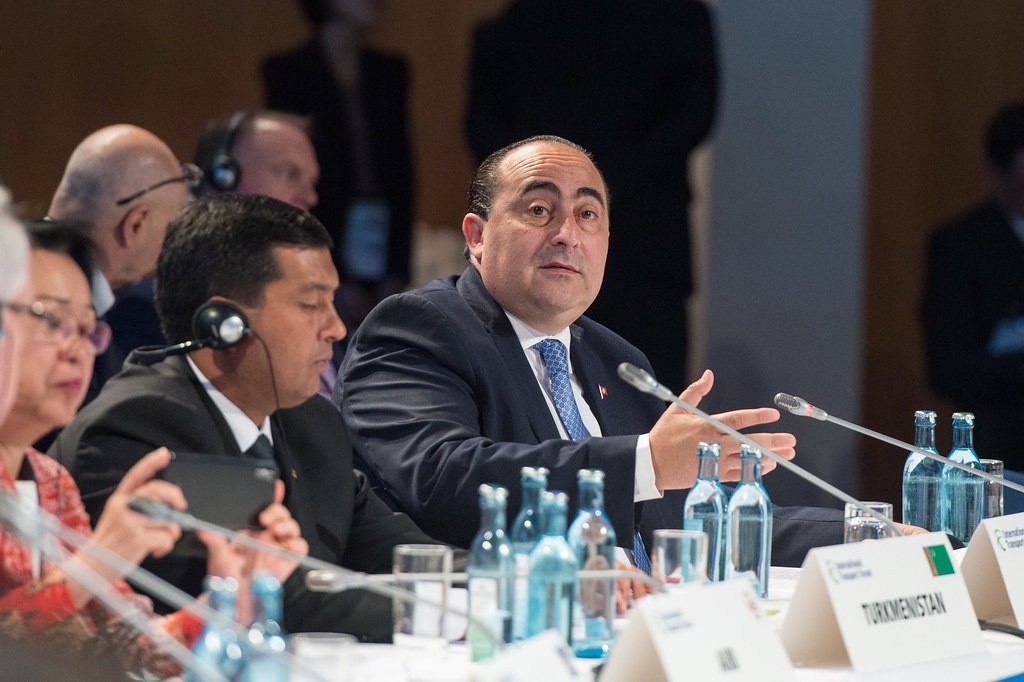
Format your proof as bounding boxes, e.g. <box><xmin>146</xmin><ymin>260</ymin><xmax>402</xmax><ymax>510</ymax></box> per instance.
<box><xmin>6</xmin><ymin>301</ymin><xmax>112</xmax><ymax>354</ymax></box>
<box><xmin>117</xmin><ymin>162</ymin><xmax>204</xmax><ymax>207</ymax></box>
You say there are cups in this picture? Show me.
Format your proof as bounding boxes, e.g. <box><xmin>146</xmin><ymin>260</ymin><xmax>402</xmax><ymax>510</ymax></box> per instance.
<box><xmin>844</xmin><ymin>501</ymin><xmax>893</xmax><ymax>543</ymax></box>
<box><xmin>652</xmin><ymin>529</ymin><xmax>709</xmax><ymax>589</ymax></box>
<box><xmin>980</xmin><ymin>459</ymin><xmax>1004</xmax><ymax>519</ymax></box>
<box><xmin>392</xmin><ymin>544</ymin><xmax>453</xmax><ymax>643</ymax></box>
<box><xmin>289</xmin><ymin>631</ymin><xmax>356</xmax><ymax>680</ymax></box>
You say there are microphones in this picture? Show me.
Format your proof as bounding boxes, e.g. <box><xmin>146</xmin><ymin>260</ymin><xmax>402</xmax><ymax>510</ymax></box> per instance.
<box><xmin>773</xmin><ymin>392</ymin><xmax>1024</xmax><ymax>494</ymax></box>
<box><xmin>617</xmin><ymin>362</ymin><xmax>908</xmax><ymax>539</ymax></box>
<box><xmin>122</xmin><ymin>496</ymin><xmax>507</xmax><ymax>651</ymax></box>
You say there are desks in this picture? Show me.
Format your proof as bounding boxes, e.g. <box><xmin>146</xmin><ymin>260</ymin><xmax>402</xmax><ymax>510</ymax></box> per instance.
<box><xmin>306</xmin><ymin>544</ymin><xmax>1024</xmax><ymax>682</ymax></box>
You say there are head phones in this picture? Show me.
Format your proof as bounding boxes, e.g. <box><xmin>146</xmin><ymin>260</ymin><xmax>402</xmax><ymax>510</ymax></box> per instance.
<box><xmin>210</xmin><ymin>107</ymin><xmax>252</xmax><ymax>191</ymax></box>
<box><xmin>131</xmin><ymin>300</ymin><xmax>252</xmax><ymax>367</ymax></box>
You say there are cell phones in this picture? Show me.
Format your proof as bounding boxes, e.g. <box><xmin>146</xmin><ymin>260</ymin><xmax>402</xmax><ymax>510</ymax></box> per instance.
<box><xmin>163</xmin><ymin>452</ymin><xmax>278</xmax><ymax>529</ymax></box>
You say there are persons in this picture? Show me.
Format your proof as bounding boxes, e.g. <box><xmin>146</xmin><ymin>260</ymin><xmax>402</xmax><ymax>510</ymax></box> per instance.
<box><xmin>919</xmin><ymin>105</ymin><xmax>1024</xmax><ymax>474</ymax></box>
<box><xmin>258</xmin><ymin>0</ymin><xmax>414</xmax><ymax>367</ymax></box>
<box><xmin>0</xmin><ymin>110</ymin><xmax>668</xmax><ymax>682</ymax></box>
<box><xmin>330</xmin><ymin>136</ymin><xmax>966</xmax><ymax>576</ymax></box>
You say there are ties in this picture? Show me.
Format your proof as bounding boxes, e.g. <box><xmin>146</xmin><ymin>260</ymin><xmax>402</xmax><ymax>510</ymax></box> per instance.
<box><xmin>533</xmin><ymin>339</ymin><xmax>651</xmax><ymax>575</ymax></box>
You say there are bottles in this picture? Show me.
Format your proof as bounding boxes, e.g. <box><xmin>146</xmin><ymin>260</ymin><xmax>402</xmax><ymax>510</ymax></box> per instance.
<box><xmin>183</xmin><ymin>574</ymin><xmax>294</xmax><ymax>682</ymax></box>
<box><xmin>941</xmin><ymin>412</ymin><xmax>985</xmax><ymax>548</ymax></box>
<box><xmin>902</xmin><ymin>409</ymin><xmax>945</xmax><ymax>532</ymax></box>
<box><xmin>528</xmin><ymin>490</ymin><xmax>582</xmax><ymax>650</ymax></box>
<box><xmin>567</xmin><ymin>468</ymin><xmax>616</xmax><ymax>658</ymax></box>
<box><xmin>725</xmin><ymin>444</ymin><xmax>773</xmax><ymax>597</ymax></box>
<box><xmin>466</xmin><ymin>482</ymin><xmax>513</xmax><ymax>660</ymax></box>
<box><xmin>682</xmin><ymin>441</ymin><xmax>729</xmax><ymax>582</ymax></box>
<box><xmin>507</xmin><ymin>466</ymin><xmax>551</xmax><ymax>643</ymax></box>
